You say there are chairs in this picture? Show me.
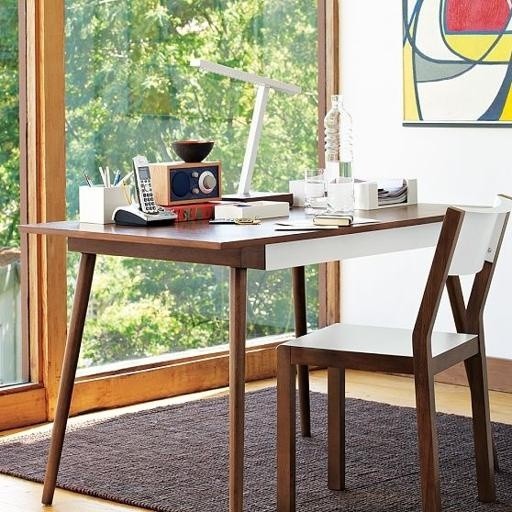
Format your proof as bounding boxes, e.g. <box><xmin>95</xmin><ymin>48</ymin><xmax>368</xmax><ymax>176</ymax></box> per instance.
<box><xmin>277</xmin><ymin>193</ymin><xmax>512</xmax><ymax>512</ymax></box>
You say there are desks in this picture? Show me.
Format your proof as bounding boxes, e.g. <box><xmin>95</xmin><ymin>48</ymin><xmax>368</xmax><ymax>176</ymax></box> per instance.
<box><xmin>17</xmin><ymin>205</ymin><xmax>499</xmax><ymax>512</ymax></box>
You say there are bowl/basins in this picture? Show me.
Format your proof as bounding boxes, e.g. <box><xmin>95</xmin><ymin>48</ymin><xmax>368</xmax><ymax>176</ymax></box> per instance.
<box><xmin>171</xmin><ymin>139</ymin><xmax>215</xmax><ymax>163</ymax></box>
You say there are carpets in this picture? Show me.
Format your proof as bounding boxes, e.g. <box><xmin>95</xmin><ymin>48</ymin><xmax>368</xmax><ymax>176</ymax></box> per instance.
<box><xmin>1</xmin><ymin>386</ymin><xmax>512</xmax><ymax>512</ymax></box>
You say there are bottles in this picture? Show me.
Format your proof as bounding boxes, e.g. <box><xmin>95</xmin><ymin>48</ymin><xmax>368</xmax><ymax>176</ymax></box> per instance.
<box><xmin>324</xmin><ymin>95</ymin><xmax>355</xmax><ymax>215</ymax></box>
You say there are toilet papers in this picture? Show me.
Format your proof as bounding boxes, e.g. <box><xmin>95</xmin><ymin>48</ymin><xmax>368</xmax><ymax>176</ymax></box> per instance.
<box><xmin>355</xmin><ymin>182</ymin><xmax>379</xmax><ymax>210</ymax></box>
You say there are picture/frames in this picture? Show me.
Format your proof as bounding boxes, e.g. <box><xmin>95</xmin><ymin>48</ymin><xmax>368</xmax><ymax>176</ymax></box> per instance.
<box><xmin>402</xmin><ymin>1</ymin><xmax>512</xmax><ymax>126</ymax></box>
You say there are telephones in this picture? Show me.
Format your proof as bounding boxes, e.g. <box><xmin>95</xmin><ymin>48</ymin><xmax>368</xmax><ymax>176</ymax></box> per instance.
<box><xmin>111</xmin><ymin>155</ymin><xmax>178</xmax><ymax>227</ymax></box>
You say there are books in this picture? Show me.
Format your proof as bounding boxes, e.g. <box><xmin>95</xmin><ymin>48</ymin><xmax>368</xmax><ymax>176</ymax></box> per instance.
<box><xmin>313</xmin><ymin>213</ymin><xmax>354</xmax><ymax>226</ymax></box>
<box><xmin>378</xmin><ymin>178</ymin><xmax>409</xmax><ymax>206</ymax></box>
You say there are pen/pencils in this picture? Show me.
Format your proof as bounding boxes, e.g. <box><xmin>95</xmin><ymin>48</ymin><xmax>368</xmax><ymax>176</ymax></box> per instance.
<box><xmin>98</xmin><ymin>166</ymin><xmax>111</xmax><ymax>188</ymax></box>
<box><xmin>113</xmin><ymin>169</ymin><xmax>120</xmax><ymax>186</ymax></box>
<box><xmin>82</xmin><ymin>172</ymin><xmax>93</xmax><ymax>189</ymax></box>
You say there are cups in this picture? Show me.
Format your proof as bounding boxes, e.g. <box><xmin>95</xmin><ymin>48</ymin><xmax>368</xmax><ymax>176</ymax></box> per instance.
<box><xmin>303</xmin><ymin>168</ymin><xmax>330</xmax><ymax>217</ymax></box>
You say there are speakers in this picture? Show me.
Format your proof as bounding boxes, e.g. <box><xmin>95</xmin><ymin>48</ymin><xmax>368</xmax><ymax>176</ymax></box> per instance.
<box><xmin>148</xmin><ymin>161</ymin><xmax>222</xmax><ymax>205</ymax></box>
